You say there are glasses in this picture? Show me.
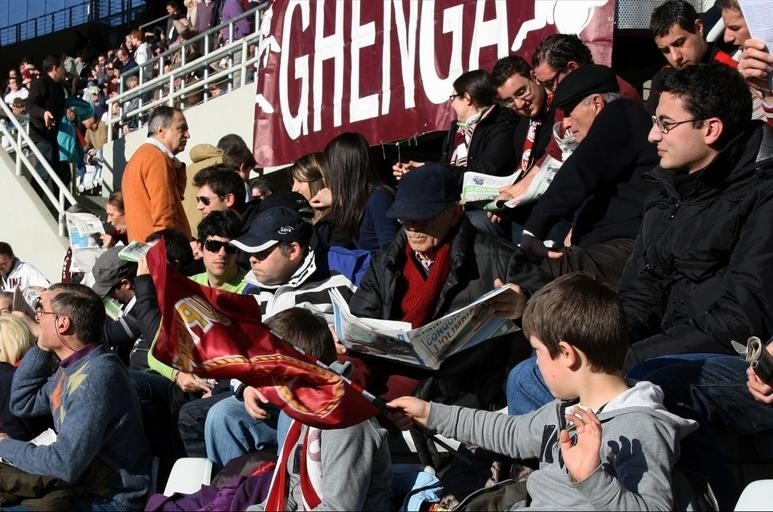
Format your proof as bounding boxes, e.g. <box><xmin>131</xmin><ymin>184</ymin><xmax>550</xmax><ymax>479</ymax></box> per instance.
<box><xmin>201</xmin><ymin>239</ymin><xmax>238</xmax><ymax>256</ymax></box>
<box><xmin>196</xmin><ymin>197</ymin><xmax>216</xmax><ymax>205</ymax></box>
<box><xmin>537</xmin><ymin>73</ymin><xmax>562</xmax><ymax>89</ymax></box>
<box><xmin>500</xmin><ymin>79</ymin><xmax>531</xmax><ymax>106</ymax></box>
<box><xmin>251</xmin><ymin>192</ymin><xmax>266</xmax><ymax>200</ymax></box>
<box><xmin>450</xmin><ymin>93</ymin><xmax>460</xmax><ymax>101</ymax></box>
<box><xmin>652</xmin><ymin>116</ymin><xmax>702</xmax><ymax>132</ymax></box>
<box><xmin>397</xmin><ymin>218</ymin><xmax>433</xmax><ymax>227</ymax></box>
<box><xmin>36</xmin><ymin>308</ymin><xmax>61</xmax><ymax>321</ymax></box>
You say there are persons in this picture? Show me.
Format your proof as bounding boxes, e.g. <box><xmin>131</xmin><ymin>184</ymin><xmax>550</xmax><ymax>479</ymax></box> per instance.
<box><xmin>115</xmin><ymin>104</ymin><xmax>197</xmax><ymax>348</ymax></box>
<box><xmin>0</xmin><ymin>0</ymin><xmax>264</xmax><ymax>183</ymax></box>
<box><xmin>357</xmin><ymin>3</ymin><xmax>773</xmax><ymax>511</ymax></box>
<box><xmin>1</xmin><ymin>137</ymin><xmax>397</xmax><ymax>511</ymax></box>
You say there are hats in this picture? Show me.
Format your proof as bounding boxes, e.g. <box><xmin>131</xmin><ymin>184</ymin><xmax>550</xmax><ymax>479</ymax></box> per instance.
<box><xmin>552</xmin><ymin>65</ymin><xmax>619</xmax><ymax>108</ymax></box>
<box><xmin>12</xmin><ymin>284</ymin><xmax>44</xmax><ymax>317</ymax></box>
<box><xmin>385</xmin><ymin>164</ymin><xmax>463</xmax><ymax>219</ymax></box>
<box><xmin>243</xmin><ymin>191</ymin><xmax>314</xmax><ymax>228</ymax></box>
<box><xmin>229</xmin><ymin>207</ymin><xmax>305</xmax><ymax>254</ymax></box>
<box><xmin>91</xmin><ymin>246</ymin><xmax>137</xmax><ymax>297</ymax></box>
